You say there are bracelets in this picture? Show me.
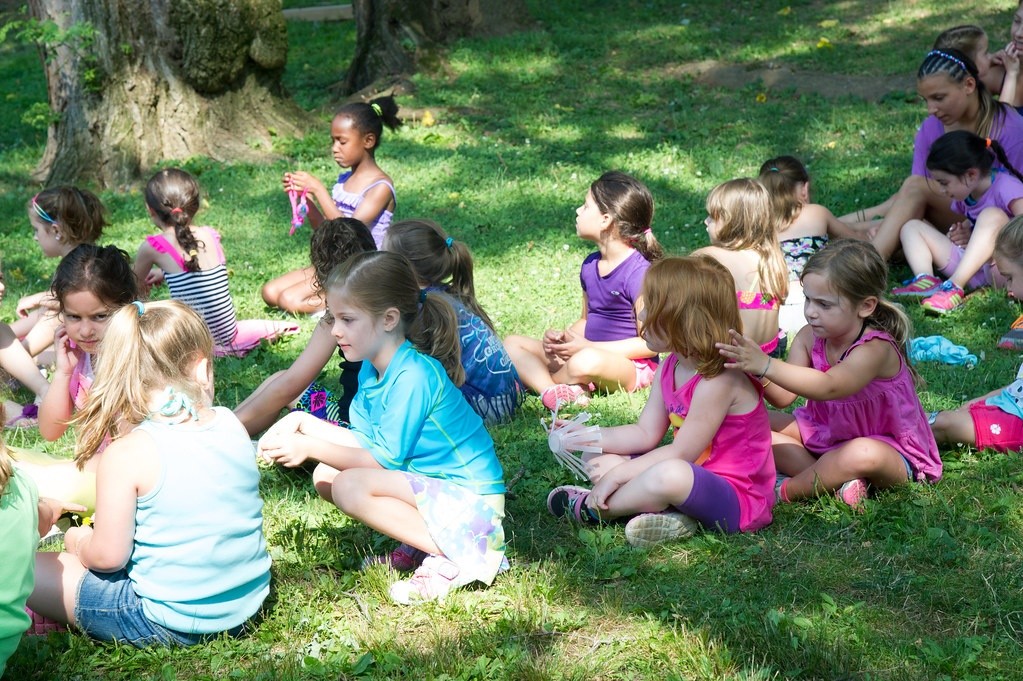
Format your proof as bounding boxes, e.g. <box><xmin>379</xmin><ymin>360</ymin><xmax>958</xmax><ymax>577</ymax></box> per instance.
<box><xmin>758</xmin><ymin>356</ymin><xmax>772</xmax><ymax>380</ymax></box>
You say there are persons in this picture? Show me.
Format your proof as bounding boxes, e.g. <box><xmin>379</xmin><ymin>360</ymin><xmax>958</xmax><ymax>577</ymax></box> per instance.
<box><xmin>502</xmin><ymin>170</ymin><xmax>662</xmax><ymax>412</ymax></box>
<box><xmin>686</xmin><ymin>155</ymin><xmax>873</xmax><ymax>358</ymax></box>
<box><xmin>0</xmin><ymin>168</ymin><xmax>240</xmax><ymax>536</ymax></box>
<box><xmin>261</xmin><ymin>95</ymin><xmax>404</xmax><ymax>313</ymax></box>
<box><xmin>548</xmin><ymin>255</ymin><xmax>776</xmax><ymax>547</ymax></box>
<box><xmin>716</xmin><ymin>236</ymin><xmax>942</xmax><ymax>512</ymax></box>
<box><xmin>233</xmin><ymin>216</ymin><xmax>526</xmax><ymax>606</ymax></box>
<box><xmin>924</xmin><ymin>215</ymin><xmax>1023</xmax><ymax>451</ymax></box>
<box><xmin>837</xmin><ymin>0</ymin><xmax>1023</xmax><ymax>317</ymax></box>
<box><xmin>0</xmin><ymin>301</ymin><xmax>271</xmax><ymax>678</ymax></box>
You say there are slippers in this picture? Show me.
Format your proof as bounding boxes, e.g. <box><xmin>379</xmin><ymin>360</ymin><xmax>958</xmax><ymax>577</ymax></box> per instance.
<box><xmin>4</xmin><ymin>403</ymin><xmax>39</xmax><ymax>429</ymax></box>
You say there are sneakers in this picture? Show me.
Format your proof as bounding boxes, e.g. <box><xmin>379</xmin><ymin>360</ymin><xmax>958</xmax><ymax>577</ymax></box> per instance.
<box><xmin>775</xmin><ymin>479</ymin><xmax>783</xmax><ymax>504</ymax></box>
<box><xmin>362</xmin><ymin>543</ymin><xmax>430</xmax><ymax>575</ymax></box>
<box><xmin>835</xmin><ymin>479</ymin><xmax>868</xmax><ymax>508</ymax></box>
<box><xmin>390</xmin><ymin>554</ymin><xmax>509</xmax><ymax>604</ymax></box>
<box><xmin>921</xmin><ymin>280</ymin><xmax>964</xmax><ymax>314</ymax></box>
<box><xmin>547</xmin><ymin>485</ymin><xmax>602</xmax><ymax>524</ymax></box>
<box><xmin>998</xmin><ymin>316</ymin><xmax>1023</xmax><ymax>350</ymax></box>
<box><xmin>890</xmin><ymin>273</ymin><xmax>941</xmax><ymax>296</ymax></box>
<box><xmin>541</xmin><ymin>385</ymin><xmax>590</xmax><ymax>412</ymax></box>
<box><xmin>625</xmin><ymin>512</ymin><xmax>698</xmax><ymax>547</ymax></box>
